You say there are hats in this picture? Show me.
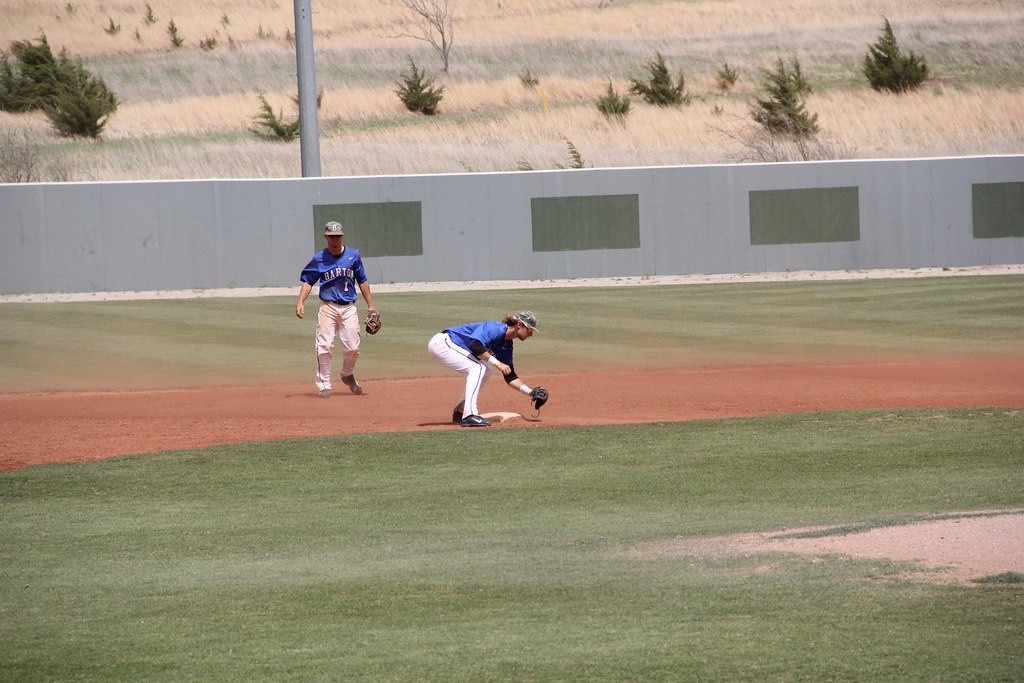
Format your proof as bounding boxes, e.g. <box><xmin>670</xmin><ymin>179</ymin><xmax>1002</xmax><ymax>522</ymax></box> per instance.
<box><xmin>514</xmin><ymin>310</ymin><xmax>539</xmax><ymax>333</ymax></box>
<box><xmin>324</xmin><ymin>221</ymin><xmax>344</xmax><ymax>235</ymax></box>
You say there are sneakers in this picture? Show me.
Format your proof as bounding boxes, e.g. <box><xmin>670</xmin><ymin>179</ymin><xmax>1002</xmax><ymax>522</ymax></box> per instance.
<box><xmin>461</xmin><ymin>415</ymin><xmax>491</xmax><ymax>427</ymax></box>
<box><xmin>452</xmin><ymin>411</ymin><xmax>463</xmax><ymax>424</ymax></box>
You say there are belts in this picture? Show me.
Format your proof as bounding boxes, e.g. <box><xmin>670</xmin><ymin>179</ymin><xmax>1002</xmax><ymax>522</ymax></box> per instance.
<box><xmin>333</xmin><ymin>301</ymin><xmax>354</xmax><ymax>305</ymax></box>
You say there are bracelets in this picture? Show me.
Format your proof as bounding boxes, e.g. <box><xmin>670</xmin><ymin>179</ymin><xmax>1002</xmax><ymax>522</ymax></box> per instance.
<box><xmin>487</xmin><ymin>355</ymin><xmax>500</xmax><ymax>368</ymax></box>
<box><xmin>520</xmin><ymin>384</ymin><xmax>532</xmax><ymax>396</ymax></box>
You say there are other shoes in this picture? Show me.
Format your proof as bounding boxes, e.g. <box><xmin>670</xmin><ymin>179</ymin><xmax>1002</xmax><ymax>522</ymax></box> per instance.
<box><xmin>341</xmin><ymin>374</ymin><xmax>362</xmax><ymax>394</ymax></box>
<box><xmin>319</xmin><ymin>389</ymin><xmax>330</xmax><ymax>398</ymax></box>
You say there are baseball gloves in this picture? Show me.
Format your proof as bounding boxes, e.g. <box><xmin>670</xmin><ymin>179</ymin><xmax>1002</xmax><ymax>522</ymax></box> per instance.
<box><xmin>530</xmin><ymin>385</ymin><xmax>549</xmax><ymax>418</ymax></box>
<box><xmin>364</xmin><ymin>309</ymin><xmax>382</xmax><ymax>337</ymax></box>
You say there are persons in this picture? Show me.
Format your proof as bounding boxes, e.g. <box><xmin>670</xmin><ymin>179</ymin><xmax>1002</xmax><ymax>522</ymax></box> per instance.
<box><xmin>428</xmin><ymin>310</ymin><xmax>549</xmax><ymax>426</ymax></box>
<box><xmin>296</xmin><ymin>221</ymin><xmax>382</xmax><ymax>398</ymax></box>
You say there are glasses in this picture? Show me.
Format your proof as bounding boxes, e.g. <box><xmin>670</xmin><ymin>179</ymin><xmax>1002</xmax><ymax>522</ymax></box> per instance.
<box><xmin>528</xmin><ymin>328</ymin><xmax>533</xmax><ymax>332</ymax></box>
